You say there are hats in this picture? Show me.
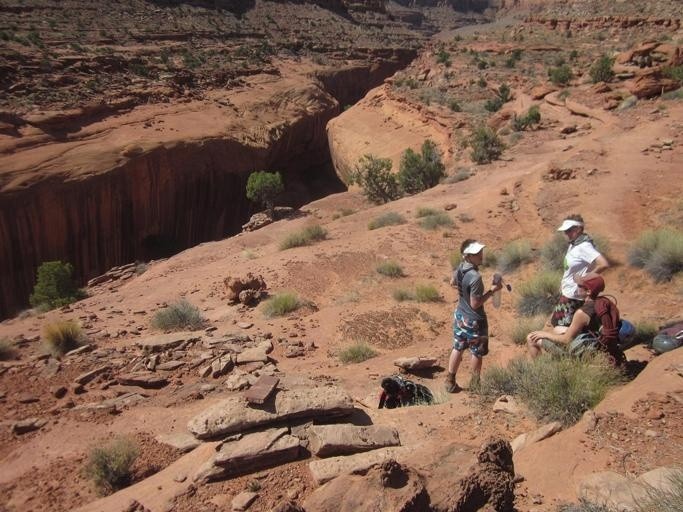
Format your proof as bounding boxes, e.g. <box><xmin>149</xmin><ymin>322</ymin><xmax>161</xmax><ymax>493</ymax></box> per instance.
<box><xmin>573</xmin><ymin>273</ymin><xmax>605</xmax><ymax>296</ymax></box>
<box><xmin>462</xmin><ymin>241</ymin><xmax>486</xmax><ymax>259</ymax></box>
<box><xmin>557</xmin><ymin>219</ymin><xmax>584</xmax><ymax>232</ymax></box>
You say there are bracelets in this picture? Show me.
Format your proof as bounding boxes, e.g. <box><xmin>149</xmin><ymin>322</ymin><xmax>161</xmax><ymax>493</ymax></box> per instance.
<box><xmin>488</xmin><ymin>289</ymin><xmax>493</xmax><ymax>296</ymax></box>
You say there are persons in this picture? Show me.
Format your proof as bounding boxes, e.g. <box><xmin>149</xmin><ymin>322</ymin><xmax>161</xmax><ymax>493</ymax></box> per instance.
<box><xmin>525</xmin><ymin>273</ymin><xmax>618</xmax><ymax>363</ymax></box>
<box><xmin>544</xmin><ymin>213</ymin><xmax>609</xmax><ymax>329</ymax></box>
<box><xmin>444</xmin><ymin>238</ymin><xmax>502</xmax><ymax>393</ymax></box>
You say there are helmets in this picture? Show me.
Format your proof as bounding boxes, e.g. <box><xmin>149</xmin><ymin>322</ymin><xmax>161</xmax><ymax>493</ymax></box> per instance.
<box><xmin>618</xmin><ymin>320</ymin><xmax>635</xmax><ymax>344</ymax></box>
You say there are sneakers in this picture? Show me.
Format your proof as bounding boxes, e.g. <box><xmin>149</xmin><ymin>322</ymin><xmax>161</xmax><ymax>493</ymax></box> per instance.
<box><xmin>445</xmin><ymin>372</ymin><xmax>463</xmax><ymax>393</ymax></box>
